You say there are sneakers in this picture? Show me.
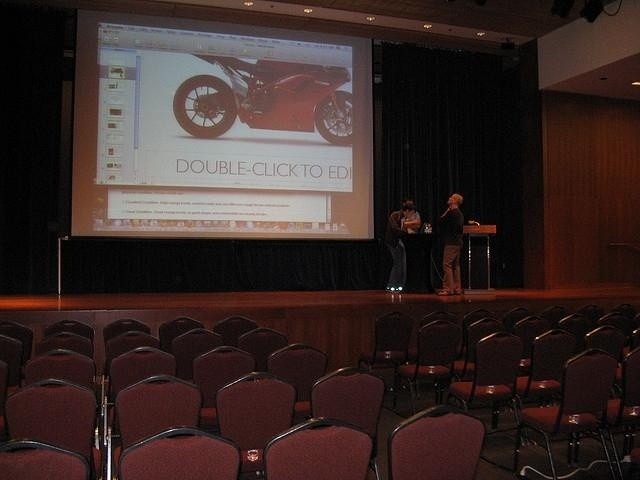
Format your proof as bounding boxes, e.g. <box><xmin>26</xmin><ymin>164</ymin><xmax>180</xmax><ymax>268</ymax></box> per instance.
<box><xmin>438</xmin><ymin>289</ymin><xmax>454</xmax><ymax>295</ymax></box>
<box><xmin>455</xmin><ymin>289</ymin><xmax>462</xmax><ymax>295</ymax></box>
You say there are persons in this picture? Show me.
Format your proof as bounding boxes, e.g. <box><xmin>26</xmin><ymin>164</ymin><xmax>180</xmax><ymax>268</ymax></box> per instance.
<box><xmin>383</xmin><ymin>199</ymin><xmax>417</xmax><ymax>295</ymax></box>
<box><xmin>437</xmin><ymin>193</ymin><xmax>464</xmax><ymax>296</ymax></box>
<box><xmin>400</xmin><ymin>200</ymin><xmax>421</xmax><ymax>234</ymax></box>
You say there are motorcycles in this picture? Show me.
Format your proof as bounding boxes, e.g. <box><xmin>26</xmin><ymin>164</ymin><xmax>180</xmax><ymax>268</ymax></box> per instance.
<box><xmin>171</xmin><ymin>53</ymin><xmax>355</xmax><ymax>146</ymax></box>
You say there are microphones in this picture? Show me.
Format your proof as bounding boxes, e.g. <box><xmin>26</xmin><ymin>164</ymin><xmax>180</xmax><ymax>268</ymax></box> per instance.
<box><xmin>468</xmin><ymin>220</ymin><xmax>479</xmax><ymax>225</ymax></box>
